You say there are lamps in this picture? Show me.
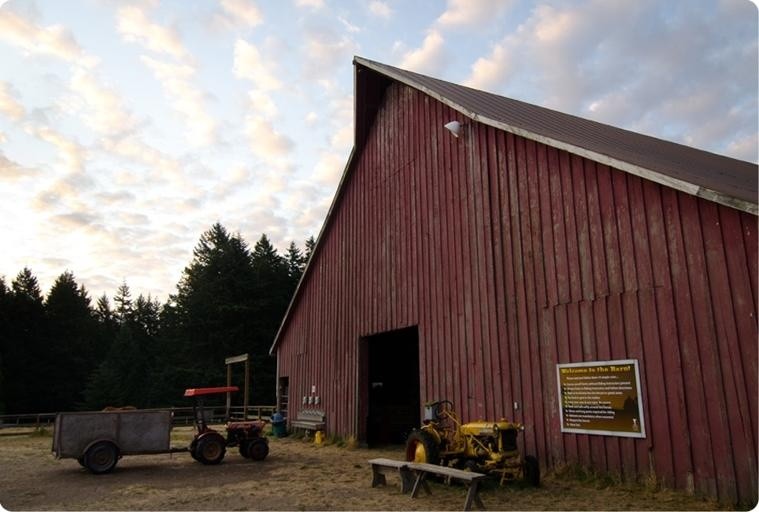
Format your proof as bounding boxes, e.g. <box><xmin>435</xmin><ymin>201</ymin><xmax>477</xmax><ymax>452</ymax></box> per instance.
<box><xmin>444</xmin><ymin>120</ymin><xmax>468</xmax><ymax>138</ymax></box>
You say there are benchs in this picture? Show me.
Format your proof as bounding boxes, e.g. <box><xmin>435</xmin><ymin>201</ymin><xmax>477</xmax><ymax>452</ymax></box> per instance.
<box><xmin>407</xmin><ymin>462</ymin><xmax>486</xmax><ymax>511</ymax></box>
<box><xmin>368</xmin><ymin>458</ymin><xmax>411</xmax><ymax>494</ymax></box>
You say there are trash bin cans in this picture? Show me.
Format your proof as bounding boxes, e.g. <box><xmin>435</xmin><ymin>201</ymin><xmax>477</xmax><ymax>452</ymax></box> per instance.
<box><xmin>270</xmin><ymin>413</ymin><xmax>286</xmax><ymax>437</ymax></box>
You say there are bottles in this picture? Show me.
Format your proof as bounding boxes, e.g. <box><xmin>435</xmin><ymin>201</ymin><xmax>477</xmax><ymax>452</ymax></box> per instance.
<box><xmin>312</xmin><ymin>384</ymin><xmax>316</xmax><ymax>393</ymax></box>
<box><xmin>309</xmin><ymin>395</ymin><xmax>313</xmax><ymax>404</ymax></box>
<box><xmin>303</xmin><ymin>395</ymin><xmax>307</xmax><ymax>404</ymax></box>
<box><xmin>315</xmin><ymin>396</ymin><xmax>319</xmax><ymax>405</ymax></box>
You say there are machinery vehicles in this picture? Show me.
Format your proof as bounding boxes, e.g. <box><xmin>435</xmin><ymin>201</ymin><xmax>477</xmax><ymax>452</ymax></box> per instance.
<box><xmin>406</xmin><ymin>401</ymin><xmax>541</xmax><ymax>487</ymax></box>
<box><xmin>51</xmin><ymin>384</ymin><xmax>270</xmax><ymax>474</ymax></box>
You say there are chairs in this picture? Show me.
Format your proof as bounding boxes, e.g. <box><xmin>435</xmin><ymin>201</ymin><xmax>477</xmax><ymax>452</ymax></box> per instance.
<box><xmin>291</xmin><ymin>409</ymin><xmax>326</xmax><ymax>440</ymax></box>
